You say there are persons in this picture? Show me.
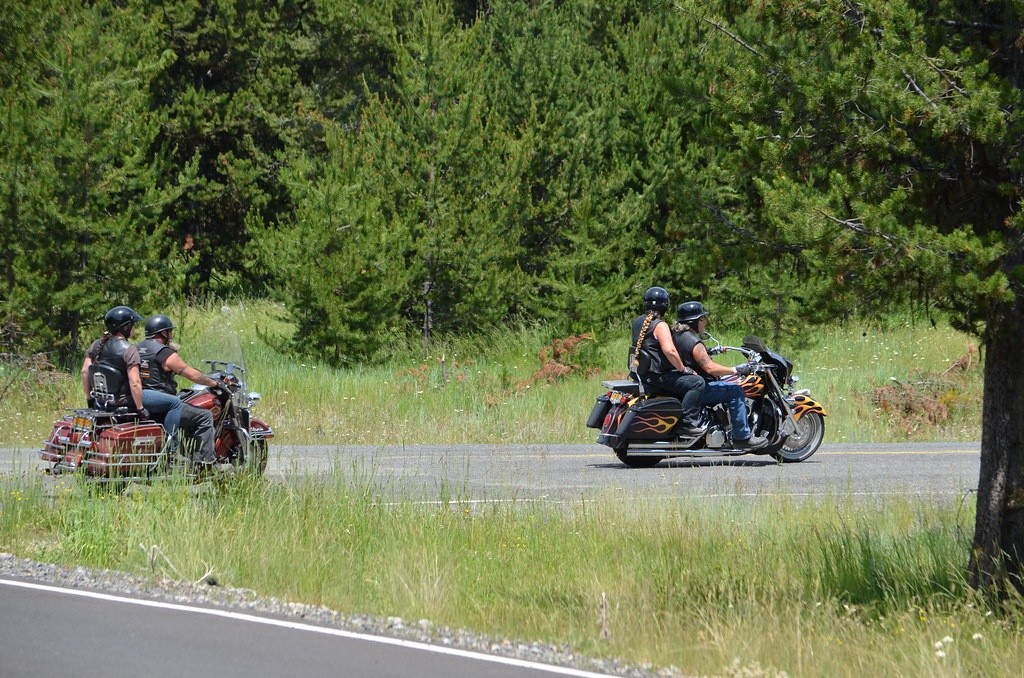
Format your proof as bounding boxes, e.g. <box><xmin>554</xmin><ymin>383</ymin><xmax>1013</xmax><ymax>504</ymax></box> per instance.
<box><xmin>671</xmin><ymin>301</ymin><xmax>769</xmax><ymax>450</ymax></box>
<box><xmin>136</xmin><ymin>314</ymin><xmax>234</xmax><ymax>476</ymax></box>
<box><xmin>630</xmin><ymin>287</ymin><xmax>704</xmax><ymax>437</ymax></box>
<box><xmin>80</xmin><ymin>305</ymin><xmax>192</xmax><ymax>465</ymax></box>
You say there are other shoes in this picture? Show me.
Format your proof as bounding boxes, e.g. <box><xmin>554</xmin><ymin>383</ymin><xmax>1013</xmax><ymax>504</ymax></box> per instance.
<box><xmin>674</xmin><ymin>423</ymin><xmax>704</xmax><ymax>436</ymax></box>
<box><xmin>171</xmin><ymin>453</ymin><xmax>191</xmax><ymax>465</ymax></box>
<box><xmin>731</xmin><ymin>436</ymin><xmax>769</xmax><ymax>450</ymax></box>
<box><xmin>204</xmin><ymin>453</ymin><xmax>217</xmax><ymax>464</ymax></box>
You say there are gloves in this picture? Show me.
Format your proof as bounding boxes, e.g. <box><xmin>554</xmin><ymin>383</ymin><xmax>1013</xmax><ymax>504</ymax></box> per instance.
<box><xmin>679</xmin><ymin>366</ymin><xmax>697</xmax><ymax>377</ymax></box>
<box><xmin>209</xmin><ymin>382</ymin><xmax>231</xmax><ymax>399</ymax></box>
<box><xmin>86</xmin><ymin>397</ymin><xmax>95</xmax><ymax>409</ymax></box>
<box><xmin>736</xmin><ymin>364</ymin><xmax>751</xmax><ymax>377</ymax></box>
<box><xmin>715</xmin><ymin>345</ymin><xmax>727</xmax><ymax>353</ymax></box>
<box><xmin>136</xmin><ymin>407</ymin><xmax>151</xmax><ymax>421</ymax></box>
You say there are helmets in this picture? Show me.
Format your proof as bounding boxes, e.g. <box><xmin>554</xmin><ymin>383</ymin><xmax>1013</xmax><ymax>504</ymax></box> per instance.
<box><xmin>143</xmin><ymin>314</ymin><xmax>174</xmax><ymax>338</ymax></box>
<box><xmin>643</xmin><ymin>286</ymin><xmax>670</xmax><ymax>307</ymax></box>
<box><xmin>676</xmin><ymin>301</ymin><xmax>708</xmax><ymax>322</ymax></box>
<box><xmin>104</xmin><ymin>306</ymin><xmax>144</xmax><ymax>332</ymax></box>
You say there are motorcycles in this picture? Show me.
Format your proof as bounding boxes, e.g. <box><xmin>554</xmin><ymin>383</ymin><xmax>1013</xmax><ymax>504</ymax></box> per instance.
<box><xmin>40</xmin><ymin>360</ymin><xmax>278</xmax><ymax>495</ymax></box>
<box><xmin>584</xmin><ymin>333</ymin><xmax>829</xmax><ymax>469</ymax></box>
